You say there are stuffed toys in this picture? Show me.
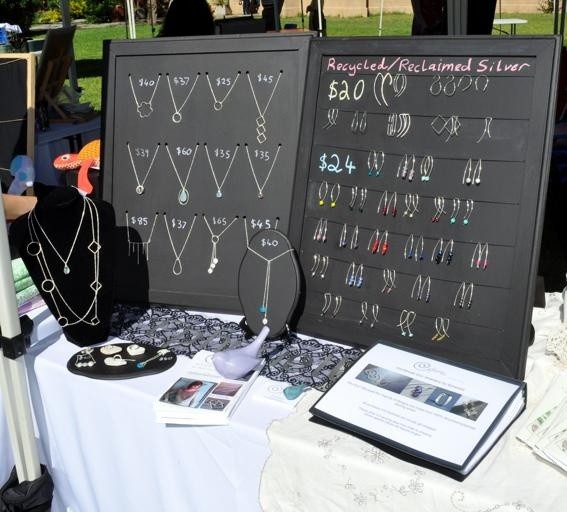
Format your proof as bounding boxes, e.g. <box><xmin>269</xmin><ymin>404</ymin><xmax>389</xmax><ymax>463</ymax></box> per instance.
<box><xmin>5</xmin><ymin>155</ymin><xmax>36</xmax><ymax>195</ymax></box>
<box><xmin>53</xmin><ymin>139</ymin><xmax>100</xmax><ymax>194</ymax></box>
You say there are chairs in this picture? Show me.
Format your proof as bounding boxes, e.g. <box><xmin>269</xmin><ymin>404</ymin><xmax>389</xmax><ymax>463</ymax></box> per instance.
<box><xmin>33</xmin><ymin>23</ymin><xmax>79</xmax><ymax>123</ymax></box>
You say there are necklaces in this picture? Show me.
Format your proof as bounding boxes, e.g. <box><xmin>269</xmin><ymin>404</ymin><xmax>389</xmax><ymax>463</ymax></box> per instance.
<box><xmin>204</xmin><ymin>144</ymin><xmax>240</xmax><ymax>198</ymax></box>
<box><xmin>32</xmin><ymin>199</ymin><xmax>87</xmax><ymax>275</ymax></box>
<box><xmin>166</xmin><ymin>74</ymin><xmax>200</xmax><ymax>123</ymax></box>
<box><xmin>125</xmin><ymin>213</ymin><xmax>159</xmax><ymax>264</ymax></box>
<box><xmin>243</xmin><ymin>219</ymin><xmax>295</xmax><ymax>326</ymax></box>
<box><xmin>206</xmin><ymin>73</ymin><xmax>240</xmax><ymax>111</ymax></box>
<box><xmin>163</xmin><ymin>214</ymin><xmax>196</xmax><ymax>275</ymax></box>
<box><xmin>25</xmin><ymin>197</ymin><xmax>101</xmax><ymax>328</ymax></box>
<box><xmin>165</xmin><ymin>144</ymin><xmax>199</xmax><ymax>206</ymax></box>
<box><xmin>246</xmin><ymin>71</ymin><xmax>282</xmax><ymax>143</ymax></box>
<box><xmin>128</xmin><ymin>74</ymin><xmax>161</xmax><ymax>118</ymax></box>
<box><xmin>245</xmin><ymin>144</ymin><xmax>281</xmax><ymax>199</ymax></box>
<box><xmin>203</xmin><ymin>215</ymin><xmax>238</xmax><ymax>274</ymax></box>
<box><xmin>126</xmin><ymin>143</ymin><xmax>160</xmax><ymax>195</ymax></box>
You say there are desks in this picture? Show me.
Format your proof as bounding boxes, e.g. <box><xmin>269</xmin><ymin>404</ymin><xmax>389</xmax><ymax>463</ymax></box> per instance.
<box><xmin>0</xmin><ymin>285</ymin><xmax>565</xmax><ymax>507</ymax></box>
<box><xmin>493</xmin><ymin>17</ymin><xmax>528</xmax><ymax>35</ymax></box>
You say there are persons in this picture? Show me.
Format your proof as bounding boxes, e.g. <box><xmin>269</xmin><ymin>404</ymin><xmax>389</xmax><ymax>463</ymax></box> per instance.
<box><xmin>147</xmin><ymin>0</ymin><xmax>327</xmax><ymax>38</ymax></box>
<box><xmin>164</xmin><ymin>377</ymin><xmax>204</xmax><ymax>408</ymax></box>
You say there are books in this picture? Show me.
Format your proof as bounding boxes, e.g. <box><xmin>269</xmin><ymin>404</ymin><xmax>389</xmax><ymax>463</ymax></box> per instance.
<box><xmin>153</xmin><ymin>349</ymin><xmax>266</xmax><ymax>426</ymax></box>
<box><xmin>309</xmin><ymin>339</ymin><xmax>527</xmax><ymax>481</ymax></box>
